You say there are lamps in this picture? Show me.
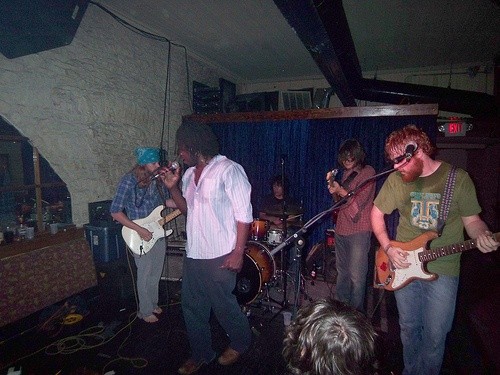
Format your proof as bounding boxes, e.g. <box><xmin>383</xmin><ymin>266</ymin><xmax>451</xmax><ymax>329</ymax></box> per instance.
<box><xmin>438</xmin><ymin>121</ymin><xmax>473</xmax><ymax>137</ymax></box>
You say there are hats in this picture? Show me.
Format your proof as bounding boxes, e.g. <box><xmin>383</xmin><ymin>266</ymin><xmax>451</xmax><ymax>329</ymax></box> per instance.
<box><xmin>135</xmin><ymin>147</ymin><xmax>161</xmax><ymax>163</ymax></box>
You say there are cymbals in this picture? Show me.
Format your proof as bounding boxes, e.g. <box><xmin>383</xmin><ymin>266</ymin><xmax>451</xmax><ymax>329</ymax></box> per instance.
<box><xmin>265</xmin><ymin>204</ymin><xmax>306</xmax><ymax>214</ymax></box>
<box><xmin>288</xmin><ymin>220</ymin><xmax>321</xmax><ymax>229</ymax></box>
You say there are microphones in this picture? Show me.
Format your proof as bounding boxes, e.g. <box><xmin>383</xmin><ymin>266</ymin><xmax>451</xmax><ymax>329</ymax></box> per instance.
<box><xmin>150</xmin><ymin>160</ymin><xmax>179</xmax><ymax>181</ymax></box>
<box><xmin>404</xmin><ymin>141</ymin><xmax>417</xmax><ymax>158</ymax></box>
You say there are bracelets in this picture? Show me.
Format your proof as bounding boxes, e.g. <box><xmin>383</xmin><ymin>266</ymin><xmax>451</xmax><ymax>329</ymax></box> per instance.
<box><xmin>233</xmin><ymin>247</ymin><xmax>244</xmax><ymax>254</ymax></box>
<box><xmin>384</xmin><ymin>244</ymin><xmax>392</xmax><ymax>252</ymax></box>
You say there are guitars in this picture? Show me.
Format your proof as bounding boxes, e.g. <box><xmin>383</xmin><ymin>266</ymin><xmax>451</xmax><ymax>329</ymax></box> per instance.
<box><xmin>375</xmin><ymin>230</ymin><xmax>500</xmax><ymax>291</ymax></box>
<box><xmin>327</xmin><ymin>169</ymin><xmax>341</xmax><ymax>224</ymax></box>
<box><xmin>121</xmin><ymin>205</ymin><xmax>182</xmax><ymax>256</ymax></box>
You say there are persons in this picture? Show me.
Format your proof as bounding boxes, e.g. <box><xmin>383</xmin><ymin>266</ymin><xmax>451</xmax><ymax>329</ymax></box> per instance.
<box><xmin>282</xmin><ymin>296</ymin><xmax>378</xmax><ymax>375</ymax></box>
<box><xmin>110</xmin><ymin>146</ymin><xmax>183</xmax><ymax>323</ymax></box>
<box><xmin>259</xmin><ymin>175</ymin><xmax>303</xmax><ymax>231</ymax></box>
<box><xmin>158</xmin><ymin>119</ymin><xmax>253</xmax><ymax>375</ymax></box>
<box><xmin>371</xmin><ymin>124</ymin><xmax>500</xmax><ymax>375</ymax></box>
<box><xmin>327</xmin><ymin>138</ymin><xmax>376</xmax><ymax>317</ymax></box>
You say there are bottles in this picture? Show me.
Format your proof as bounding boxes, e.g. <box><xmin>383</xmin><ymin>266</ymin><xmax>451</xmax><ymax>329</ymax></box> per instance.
<box><xmin>311</xmin><ymin>264</ymin><xmax>317</xmax><ymax>278</ymax></box>
<box><xmin>15</xmin><ymin>210</ymin><xmax>58</xmax><ymax>241</ymax></box>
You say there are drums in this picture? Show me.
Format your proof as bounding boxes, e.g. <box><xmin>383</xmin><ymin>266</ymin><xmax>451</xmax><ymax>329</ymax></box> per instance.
<box><xmin>247</xmin><ymin>218</ymin><xmax>270</xmax><ymax>240</ymax></box>
<box><xmin>263</xmin><ymin>230</ymin><xmax>292</xmax><ymax>246</ymax></box>
<box><xmin>231</xmin><ymin>241</ymin><xmax>276</xmax><ymax>306</ymax></box>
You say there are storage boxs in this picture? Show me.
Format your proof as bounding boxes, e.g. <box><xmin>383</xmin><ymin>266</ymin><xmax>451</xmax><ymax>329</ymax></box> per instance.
<box><xmin>83</xmin><ymin>223</ymin><xmax>125</xmax><ymax>263</ymax></box>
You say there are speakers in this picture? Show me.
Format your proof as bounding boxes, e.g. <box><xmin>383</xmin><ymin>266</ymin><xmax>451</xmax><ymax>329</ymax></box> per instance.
<box><xmin>325</xmin><ymin>229</ymin><xmax>338</xmax><ymax>284</ymax></box>
<box><xmin>88</xmin><ymin>200</ymin><xmax>127</xmax><ymax>226</ymax></box>
<box><xmin>0</xmin><ymin>0</ymin><xmax>88</xmax><ymax>59</ymax></box>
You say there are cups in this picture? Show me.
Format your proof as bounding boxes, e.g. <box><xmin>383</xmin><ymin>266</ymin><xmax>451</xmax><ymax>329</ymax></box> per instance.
<box><xmin>283</xmin><ymin>312</ymin><xmax>291</xmax><ymax>326</ymax></box>
<box><xmin>3</xmin><ymin>228</ymin><xmax>14</xmax><ymax>242</ymax></box>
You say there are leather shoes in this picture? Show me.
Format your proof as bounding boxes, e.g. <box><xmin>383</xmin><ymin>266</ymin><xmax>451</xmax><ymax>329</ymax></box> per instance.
<box><xmin>178</xmin><ymin>356</ymin><xmax>205</xmax><ymax>374</ymax></box>
<box><xmin>218</xmin><ymin>346</ymin><xmax>241</xmax><ymax>365</ymax></box>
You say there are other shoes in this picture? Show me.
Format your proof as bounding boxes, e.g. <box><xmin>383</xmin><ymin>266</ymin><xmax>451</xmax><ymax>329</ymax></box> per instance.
<box><xmin>138</xmin><ymin>313</ymin><xmax>158</xmax><ymax>323</ymax></box>
<box><xmin>153</xmin><ymin>305</ymin><xmax>162</xmax><ymax>313</ymax></box>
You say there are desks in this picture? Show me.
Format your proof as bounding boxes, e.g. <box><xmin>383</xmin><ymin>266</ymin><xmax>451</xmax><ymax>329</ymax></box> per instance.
<box><xmin>0</xmin><ymin>228</ymin><xmax>98</xmax><ymax>328</ymax></box>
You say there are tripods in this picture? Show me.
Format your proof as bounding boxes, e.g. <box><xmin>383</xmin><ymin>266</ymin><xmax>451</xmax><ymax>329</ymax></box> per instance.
<box><xmin>244</xmin><ymin>162</ymin><xmax>312</xmax><ymax>322</ymax></box>
